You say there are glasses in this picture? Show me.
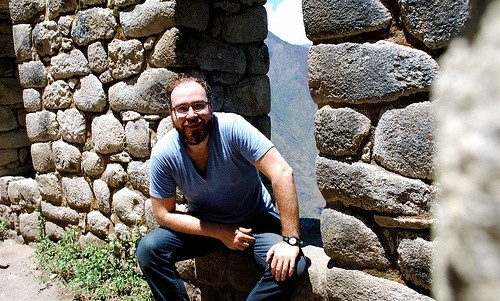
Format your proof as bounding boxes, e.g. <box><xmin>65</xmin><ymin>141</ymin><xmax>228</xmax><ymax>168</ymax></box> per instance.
<box><xmin>170</xmin><ymin>101</ymin><xmax>213</xmax><ymax>113</ymax></box>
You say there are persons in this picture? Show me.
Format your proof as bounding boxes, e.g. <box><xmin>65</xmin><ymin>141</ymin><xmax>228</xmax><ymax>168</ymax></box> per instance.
<box><xmin>136</xmin><ymin>72</ymin><xmax>306</xmax><ymax>301</ymax></box>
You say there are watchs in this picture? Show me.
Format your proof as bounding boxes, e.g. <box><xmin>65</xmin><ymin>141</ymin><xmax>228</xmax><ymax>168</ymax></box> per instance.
<box><xmin>283</xmin><ymin>235</ymin><xmax>303</xmax><ymax>246</ymax></box>
